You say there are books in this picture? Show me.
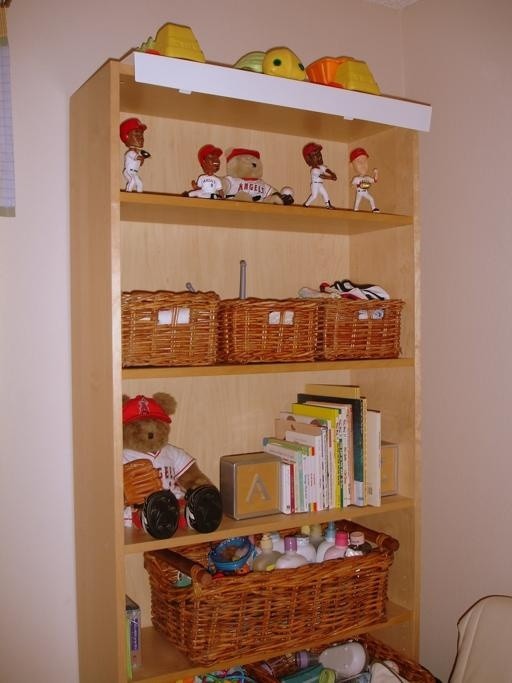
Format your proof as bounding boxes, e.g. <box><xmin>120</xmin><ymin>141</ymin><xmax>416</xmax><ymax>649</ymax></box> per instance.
<box><xmin>262</xmin><ymin>378</ymin><xmax>385</xmax><ymax>515</ymax></box>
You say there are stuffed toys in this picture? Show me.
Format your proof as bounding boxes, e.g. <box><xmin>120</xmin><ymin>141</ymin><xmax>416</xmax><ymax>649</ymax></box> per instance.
<box><xmin>220</xmin><ymin>148</ymin><xmax>295</xmax><ymax>205</ymax></box>
<box><xmin>121</xmin><ymin>391</ymin><xmax>223</xmax><ymax>536</ymax></box>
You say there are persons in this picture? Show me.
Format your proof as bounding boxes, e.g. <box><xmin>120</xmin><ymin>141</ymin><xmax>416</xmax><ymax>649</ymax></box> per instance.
<box><xmin>349</xmin><ymin>148</ymin><xmax>379</xmax><ymax>211</ymax></box>
<box><xmin>302</xmin><ymin>143</ymin><xmax>337</xmax><ymax>208</ymax></box>
<box><xmin>181</xmin><ymin>144</ymin><xmax>226</xmax><ymax>199</ymax></box>
<box><xmin>120</xmin><ymin>118</ymin><xmax>148</xmax><ymax>193</ymax></box>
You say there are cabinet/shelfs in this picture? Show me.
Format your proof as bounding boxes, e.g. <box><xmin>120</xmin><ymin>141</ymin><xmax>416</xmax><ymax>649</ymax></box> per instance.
<box><xmin>68</xmin><ymin>48</ymin><xmax>433</xmax><ymax>680</ymax></box>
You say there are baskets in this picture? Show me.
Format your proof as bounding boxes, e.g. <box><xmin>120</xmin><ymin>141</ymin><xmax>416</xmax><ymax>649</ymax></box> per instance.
<box><xmin>121</xmin><ymin>290</ymin><xmax>220</xmax><ymax>368</ymax></box>
<box><xmin>219</xmin><ymin>297</ymin><xmax>325</xmax><ymax>365</ymax></box>
<box><xmin>314</xmin><ymin>298</ymin><xmax>406</xmax><ymax>362</ymax></box>
<box><xmin>242</xmin><ymin>632</ymin><xmax>437</xmax><ymax>683</ymax></box>
<box><xmin>142</xmin><ymin>519</ymin><xmax>400</xmax><ymax>668</ymax></box>
